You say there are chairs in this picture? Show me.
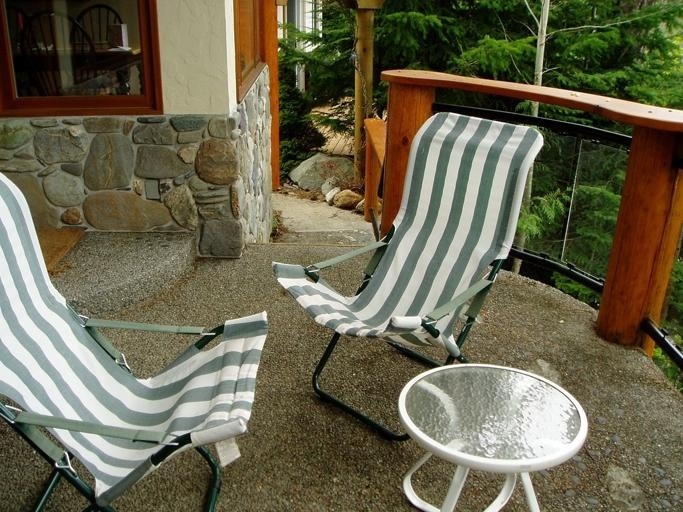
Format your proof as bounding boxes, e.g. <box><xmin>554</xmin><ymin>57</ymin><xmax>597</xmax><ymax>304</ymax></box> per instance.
<box><xmin>71</xmin><ymin>3</ymin><xmax>126</xmax><ymax>47</ymax></box>
<box><xmin>270</xmin><ymin>112</ymin><xmax>544</xmax><ymax>441</ymax></box>
<box><xmin>13</xmin><ymin>10</ymin><xmax>125</xmax><ymax>94</ymax></box>
<box><xmin>1</xmin><ymin>172</ymin><xmax>269</xmax><ymax>512</ymax></box>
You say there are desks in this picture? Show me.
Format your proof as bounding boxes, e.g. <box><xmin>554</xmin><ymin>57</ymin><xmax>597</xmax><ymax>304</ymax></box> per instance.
<box><xmin>12</xmin><ymin>42</ymin><xmax>144</xmax><ymax>97</ymax></box>
